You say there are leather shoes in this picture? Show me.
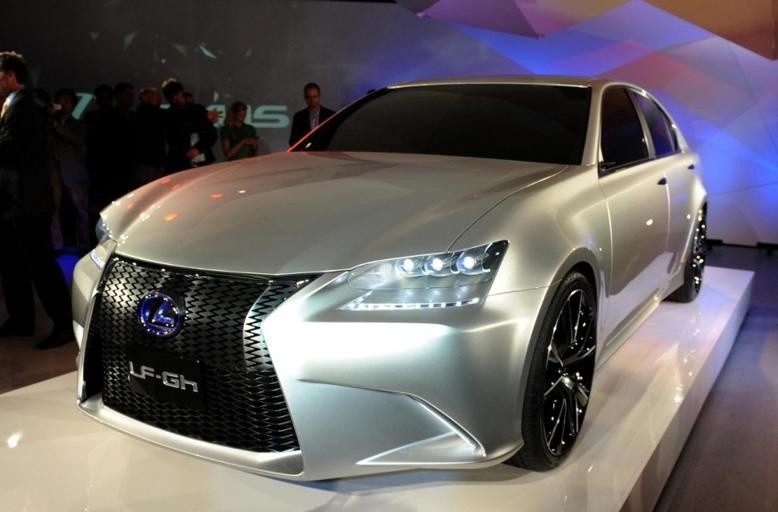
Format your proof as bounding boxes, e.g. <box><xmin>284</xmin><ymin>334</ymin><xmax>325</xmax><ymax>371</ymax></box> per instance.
<box><xmin>38</xmin><ymin>327</ymin><xmax>75</xmax><ymax>349</ymax></box>
<box><xmin>0</xmin><ymin>320</ymin><xmax>37</xmax><ymax>335</ymax></box>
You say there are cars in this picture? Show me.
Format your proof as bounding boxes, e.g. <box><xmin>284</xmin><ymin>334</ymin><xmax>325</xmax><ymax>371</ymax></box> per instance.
<box><xmin>70</xmin><ymin>75</ymin><xmax>710</xmax><ymax>483</ymax></box>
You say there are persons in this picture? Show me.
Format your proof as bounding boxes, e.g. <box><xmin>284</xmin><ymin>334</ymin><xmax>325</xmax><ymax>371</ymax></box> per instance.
<box><xmin>288</xmin><ymin>81</ymin><xmax>336</xmax><ymax>148</ymax></box>
